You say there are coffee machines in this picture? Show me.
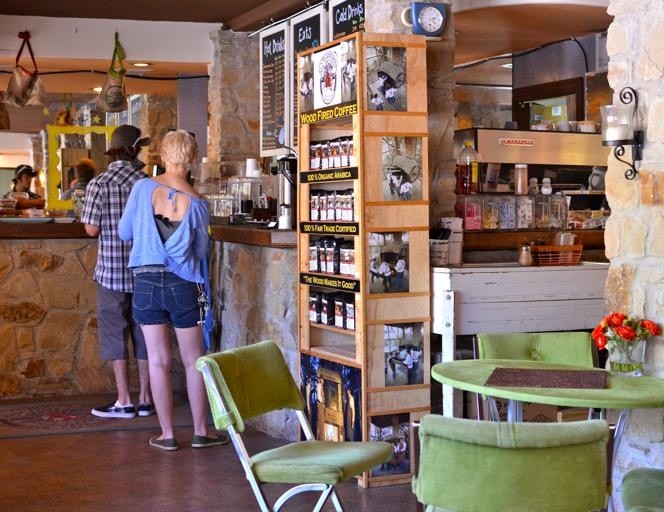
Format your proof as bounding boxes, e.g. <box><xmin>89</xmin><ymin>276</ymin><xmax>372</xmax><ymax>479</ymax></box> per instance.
<box><xmin>269</xmin><ymin>145</ymin><xmax>297</xmax><ymax>231</ymax></box>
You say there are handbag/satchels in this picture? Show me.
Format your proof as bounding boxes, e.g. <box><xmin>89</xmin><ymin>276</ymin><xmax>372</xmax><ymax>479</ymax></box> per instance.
<box><xmin>96</xmin><ymin>69</ymin><xmax>128</xmax><ymax>112</ymax></box>
<box><xmin>2</xmin><ymin>65</ymin><xmax>46</xmax><ymax>108</ymax></box>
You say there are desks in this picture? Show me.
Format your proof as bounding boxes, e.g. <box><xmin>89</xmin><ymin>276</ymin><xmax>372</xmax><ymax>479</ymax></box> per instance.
<box><xmin>430</xmin><ymin>356</ymin><xmax>662</xmax><ymax>424</ymax></box>
<box><xmin>430</xmin><ymin>263</ymin><xmax>611</xmax><ymax>414</ymax></box>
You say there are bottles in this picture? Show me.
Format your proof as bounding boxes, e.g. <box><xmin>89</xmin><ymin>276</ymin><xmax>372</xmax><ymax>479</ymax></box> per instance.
<box><xmin>458</xmin><ymin>140</ymin><xmax>480</xmax><ymax>195</ymax></box>
<box><xmin>517</xmin><ymin>242</ymin><xmax>534</xmax><ymax>266</ymax></box>
<box><xmin>529</xmin><ymin>176</ymin><xmax>554</xmax><ymax>196</ymax></box>
<box><xmin>513</xmin><ymin>163</ymin><xmax>528</xmax><ymax>195</ymax></box>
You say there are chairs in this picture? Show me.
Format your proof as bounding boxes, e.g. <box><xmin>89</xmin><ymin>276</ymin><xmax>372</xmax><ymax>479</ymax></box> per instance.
<box><xmin>408</xmin><ymin>413</ymin><xmax>612</xmax><ymax>510</ymax></box>
<box><xmin>475</xmin><ymin>329</ymin><xmax>592</xmax><ymax>370</ymax></box>
<box><xmin>619</xmin><ymin>464</ymin><xmax>663</xmax><ymax>512</ymax></box>
<box><xmin>191</xmin><ymin>339</ymin><xmax>395</xmax><ymax>510</ymax></box>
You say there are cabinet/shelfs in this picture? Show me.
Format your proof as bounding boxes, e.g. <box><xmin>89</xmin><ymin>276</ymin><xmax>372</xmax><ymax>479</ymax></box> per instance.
<box><xmin>294</xmin><ymin>38</ymin><xmax>432</xmax><ymax>490</ymax></box>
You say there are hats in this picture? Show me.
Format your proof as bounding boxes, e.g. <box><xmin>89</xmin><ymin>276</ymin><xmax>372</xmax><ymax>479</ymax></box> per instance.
<box><xmin>15</xmin><ymin>165</ymin><xmax>37</xmax><ymax>176</ymax></box>
<box><xmin>111</xmin><ymin>124</ymin><xmax>150</xmax><ymax>145</ymax></box>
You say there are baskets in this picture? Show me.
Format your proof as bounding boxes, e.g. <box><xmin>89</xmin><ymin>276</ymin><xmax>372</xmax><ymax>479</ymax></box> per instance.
<box><xmin>518</xmin><ymin>236</ymin><xmax>583</xmax><ymax>266</ymax></box>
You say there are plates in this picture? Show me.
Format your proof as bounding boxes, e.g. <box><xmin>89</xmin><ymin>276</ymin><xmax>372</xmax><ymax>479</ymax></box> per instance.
<box><xmin>54</xmin><ymin>216</ymin><xmax>76</xmax><ymax>224</ymax></box>
<box><xmin>0</xmin><ymin>217</ymin><xmax>53</xmax><ymax>223</ymax></box>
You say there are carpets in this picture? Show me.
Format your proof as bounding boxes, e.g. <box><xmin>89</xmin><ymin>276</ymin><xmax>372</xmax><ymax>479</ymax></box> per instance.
<box><xmin>0</xmin><ymin>394</ymin><xmax>234</xmax><ymax>440</ymax></box>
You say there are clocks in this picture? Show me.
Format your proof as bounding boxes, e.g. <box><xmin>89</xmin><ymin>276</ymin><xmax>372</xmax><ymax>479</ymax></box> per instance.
<box><xmin>400</xmin><ymin>2</ymin><xmax>449</xmax><ymax>41</ymax></box>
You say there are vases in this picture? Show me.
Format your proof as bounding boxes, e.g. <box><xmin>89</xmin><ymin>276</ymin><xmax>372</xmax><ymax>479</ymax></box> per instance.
<box><xmin>591</xmin><ymin>310</ymin><xmax>662</xmax><ymax>373</ymax></box>
<box><xmin>607</xmin><ymin>338</ymin><xmax>647</xmax><ymax>377</ymax></box>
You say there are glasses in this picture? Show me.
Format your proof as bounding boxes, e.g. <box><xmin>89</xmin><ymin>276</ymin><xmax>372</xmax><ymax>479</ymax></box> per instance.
<box><xmin>168</xmin><ymin>127</ymin><xmax>195</xmax><ymax>138</ymax></box>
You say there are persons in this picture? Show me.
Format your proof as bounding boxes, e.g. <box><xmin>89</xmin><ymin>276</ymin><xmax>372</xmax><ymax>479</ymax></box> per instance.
<box><xmin>302</xmin><ymin>373</ymin><xmax>356</xmax><ymax>442</ymax></box>
<box><xmin>299</xmin><ymin>51</ymin><xmax>359</xmax><ymax>108</ymax></box>
<box><xmin>79</xmin><ymin>124</ymin><xmax>162</xmax><ymax>420</ymax></box>
<box><xmin>118</xmin><ymin>126</ymin><xmax>230</xmax><ymax>453</ymax></box>
<box><xmin>57</xmin><ymin>167</ymin><xmax>76</xmax><ymax>218</ymax></box>
<box><xmin>367</xmin><ymin>69</ymin><xmax>424</xmax><ymax>477</ymax></box>
<box><xmin>70</xmin><ymin>157</ymin><xmax>98</xmax><ymax>197</ymax></box>
<box><xmin>4</xmin><ymin>163</ymin><xmax>45</xmax><ymax>209</ymax></box>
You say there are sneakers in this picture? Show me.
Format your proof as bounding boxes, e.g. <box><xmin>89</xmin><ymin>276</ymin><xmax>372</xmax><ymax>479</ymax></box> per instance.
<box><xmin>138</xmin><ymin>403</ymin><xmax>156</xmax><ymax>417</ymax></box>
<box><xmin>191</xmin><ymin>435</ymin><xmax>229</xmax><ymax>448</ymax></box>
<box><xmin>149</xmin><ymin>436</ymin><xmax>179</xmax><ymax>451</ymax></box>
<box><xmin>91</xmin><ymin>401</ymin><xmax>136</xmax><ymax>419</ymax></box>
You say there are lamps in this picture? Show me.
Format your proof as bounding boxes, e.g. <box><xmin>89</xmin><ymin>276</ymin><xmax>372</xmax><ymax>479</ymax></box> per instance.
<box><xmin>599</xmin><ymin>84</ymin><xmax>648</xmax><ymax>180</ymax></box>
